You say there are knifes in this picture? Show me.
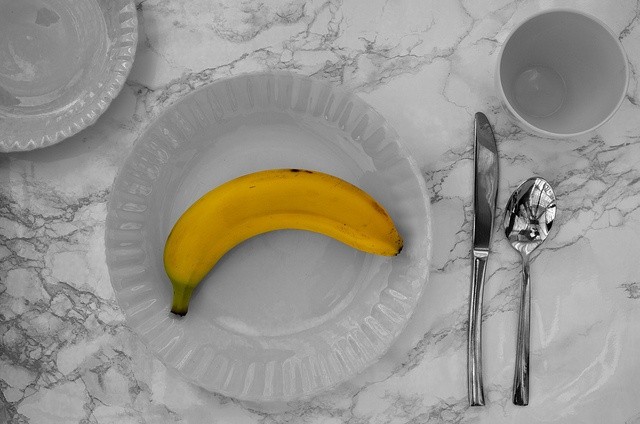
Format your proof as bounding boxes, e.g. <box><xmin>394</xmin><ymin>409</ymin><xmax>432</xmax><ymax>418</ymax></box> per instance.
<box><xmin>468</xmin><ymin>112</ymin><xmax>497</xmax><ymax>406</ymax></box>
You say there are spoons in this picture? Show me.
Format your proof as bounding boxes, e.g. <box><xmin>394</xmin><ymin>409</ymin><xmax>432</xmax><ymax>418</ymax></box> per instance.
<box><xmin>503</xmin><ymin>177</ymin><xmax>556</xmax><ymax>405</ymax></box>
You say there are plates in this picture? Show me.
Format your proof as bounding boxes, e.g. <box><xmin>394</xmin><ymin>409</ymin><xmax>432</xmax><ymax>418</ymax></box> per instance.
<box><xmin>0</xmin><ymin>0</ymin><xmax>138</xmax><ymax>154</ymax></box>
<box><xmin>105</xmin><ymin>74</ymin><xmax>432</xmax><ymax>404</ymax></box>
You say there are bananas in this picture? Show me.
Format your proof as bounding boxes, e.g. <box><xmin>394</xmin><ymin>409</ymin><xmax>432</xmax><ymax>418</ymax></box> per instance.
<box><xmin>163</xmin><ymin>168</ymin><xmax>405</xmax><ymax>317</ymax></box>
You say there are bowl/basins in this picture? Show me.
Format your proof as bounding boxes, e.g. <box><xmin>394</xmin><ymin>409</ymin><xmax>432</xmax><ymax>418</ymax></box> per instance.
<box><xmin>497</xmin><ymin>8</ymin><xmax>628</xmax><ymax>138</ymax></box>
